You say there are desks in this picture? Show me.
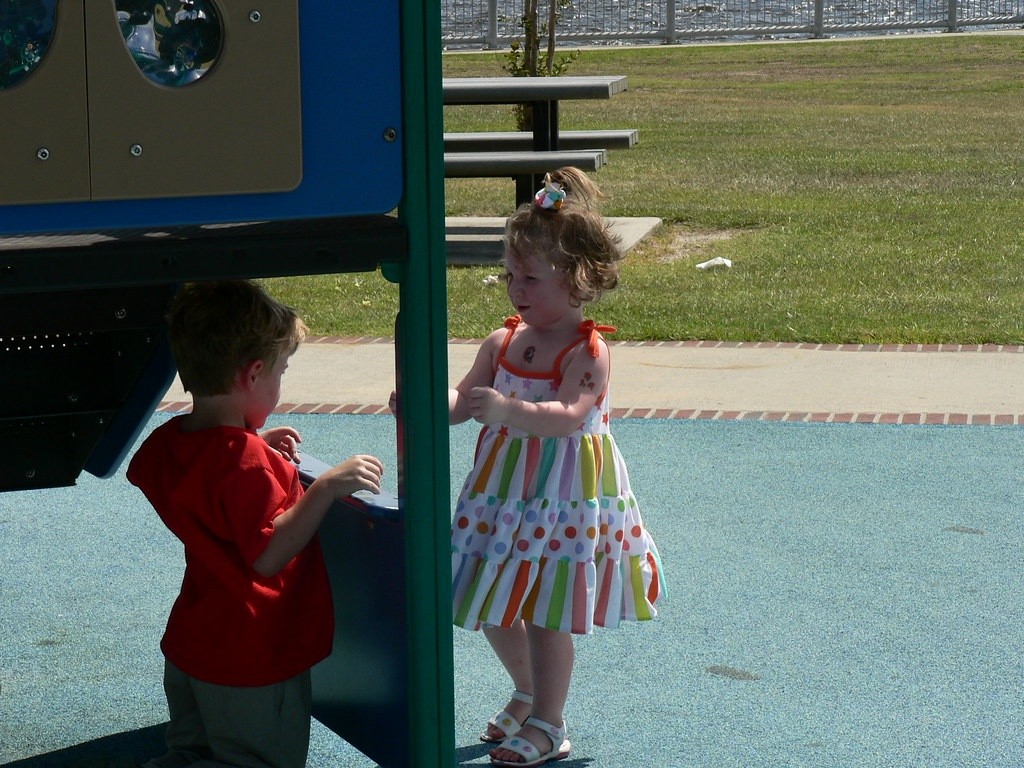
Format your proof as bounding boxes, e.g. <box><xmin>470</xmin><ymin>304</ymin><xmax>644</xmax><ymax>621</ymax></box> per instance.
<box><xmin>443</xmin><ymin>75</ymin><xmax>628</xmax><ymax>204</ymax></box>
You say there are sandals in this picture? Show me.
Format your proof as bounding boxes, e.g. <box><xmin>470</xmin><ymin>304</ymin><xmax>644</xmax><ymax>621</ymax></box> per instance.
<box><xmin>481</xmin><ymin>690</ymin><xmax>534</xmax><ymax>741</ymax></box>
<box><xmin>490</xmin><ymin>714</ymin><xmax>571</xmax><ymax>767</ymax></box>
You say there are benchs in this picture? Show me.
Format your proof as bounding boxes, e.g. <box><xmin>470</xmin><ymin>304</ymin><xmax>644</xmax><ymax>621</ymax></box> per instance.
<box><xmin>443</xmin><ymin>130</ymin><xmax>639</xmax><ymax>211</ymax></box>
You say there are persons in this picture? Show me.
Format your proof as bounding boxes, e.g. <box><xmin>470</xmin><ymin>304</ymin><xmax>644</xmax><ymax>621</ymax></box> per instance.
<box><xmin>388</xmin><ymin>168</ymin><xmax>669</xmax><ymax>767</ymax></box>
<box><xmin>125</xmin><ymin>280</ymin><xmax>383</xmax><ymax>768</ymax></box>
